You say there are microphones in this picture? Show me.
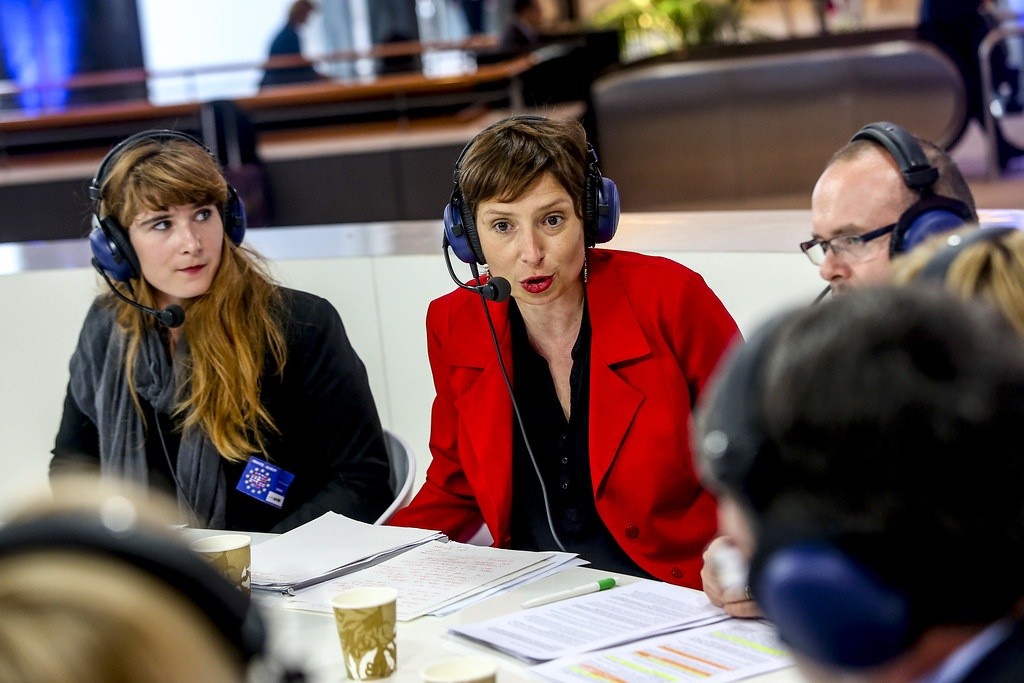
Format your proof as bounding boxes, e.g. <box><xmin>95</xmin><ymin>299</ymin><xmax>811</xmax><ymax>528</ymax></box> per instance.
<box><xmin>102</xmin><ymin>274</ymin><xmax>186</xmax><ymax>328</ymax></box>
<box><xmin>442</xmin><ymin>247</ymin><xmax>511</xmax><ymax>302</ymax></box>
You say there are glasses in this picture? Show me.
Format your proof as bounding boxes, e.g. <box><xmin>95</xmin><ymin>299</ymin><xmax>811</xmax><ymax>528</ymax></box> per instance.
<box><xmin>800</xmin><ymin>223</ymin><xmax>896</xmax><ymax>267</ymax></box>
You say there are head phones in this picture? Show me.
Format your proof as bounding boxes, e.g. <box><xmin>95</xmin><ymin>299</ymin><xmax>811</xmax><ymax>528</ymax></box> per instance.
<box><xmin>443</xmin><ymin>114</ymin><xmax>622</xmax><ymax>265</ymax></box>
<box><xmin>88</xmin><ymin>129</ymin><xmax>246</xmax><ymax>282</ymax></box>
<box><xmin>703</xmin><ymin>308</ymin><xmax>922</xmax><ymax>672</ymax></box>
<box><xmin>851</xmin><ymin>120</ymin><xmax>977</xmax><ymax>262</ymax></box>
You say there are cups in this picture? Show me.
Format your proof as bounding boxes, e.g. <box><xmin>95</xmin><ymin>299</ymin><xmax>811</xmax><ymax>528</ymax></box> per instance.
<box><xmin>189</xmin><ymin>534</ymin><xmax>252</xmax><ymax>599</ymax></box>
<box><xmin>329</xmin><ymin>585</ymin><xmax>398</xmax><ymax>681</ymax></box>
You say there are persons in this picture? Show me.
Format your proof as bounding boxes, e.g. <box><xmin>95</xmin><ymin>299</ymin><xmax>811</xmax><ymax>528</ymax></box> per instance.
<box><xmin>387</xmin><ymin>116</ymin><xmax>745</xmax><ymax>592</ymax></box>
<box><xmin>916</xmin><ymin>0</ymin><xmax>1024</xmax><ymax>172</ymax></box>
<box><xmin>0</xmin><ymin>471</ymin><xmax>273</xmax><ymax>683</ymax></box>
<box><xmin>693</xmin><ymin>119</ymin><xmax>1024</xmax><ymax>682</ymax></box>
<box><xmin>259</xmin><ymin>0</ymin><xmax>328</xmax><ymax>86</ymax></box>
<box><xmin>47</xmin><ymin>127</ymin><xmax>393</xmax><ymax>533</ymax></box>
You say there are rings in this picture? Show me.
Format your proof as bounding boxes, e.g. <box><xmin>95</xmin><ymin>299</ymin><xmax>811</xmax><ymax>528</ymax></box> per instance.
<box><xmin>745</xmin><ymin>584</ymin><xmax>752</xmax><ymax>600</ymax></box>
